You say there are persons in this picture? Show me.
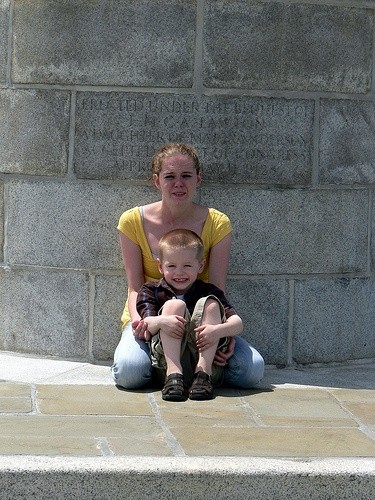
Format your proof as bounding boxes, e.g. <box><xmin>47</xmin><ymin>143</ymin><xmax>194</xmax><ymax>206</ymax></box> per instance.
<box><xmin>136</xmin><ymin>229</ymin><xmax>243</xmax><ymax>401</ymax></box>
<box><xmin>111</xmin><ymin>143</ymin><xmax>265</xmax><ymax>389</ymax></box>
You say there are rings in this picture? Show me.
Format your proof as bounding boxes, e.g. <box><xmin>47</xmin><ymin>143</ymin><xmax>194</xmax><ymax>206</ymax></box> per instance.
<box><xmin>224</xmin><ymin>360</ymin><xmax>228</xmax><ymax>366</ymax></box>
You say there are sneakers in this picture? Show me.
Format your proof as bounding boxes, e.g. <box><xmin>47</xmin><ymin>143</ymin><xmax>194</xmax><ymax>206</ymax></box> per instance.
<box><xmin>189</xmin><ymin>371</ymin><xmax>215</xmax><ymax>400</ymax></box>
<box><xmin>162</xmin><ymin>373</ymin><xmax>188</xmax><ymax>401</ymax></box>
